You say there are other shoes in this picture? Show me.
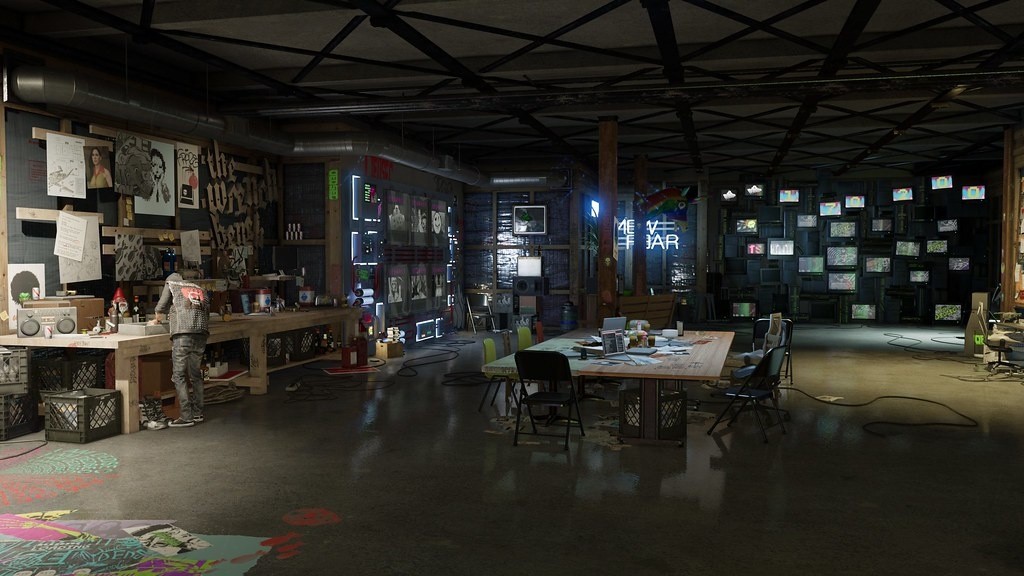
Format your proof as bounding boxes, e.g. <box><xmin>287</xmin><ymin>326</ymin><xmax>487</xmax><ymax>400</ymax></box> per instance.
<box><xmin>193</xmin><ymin>415</ymin><xmax>204</xmax><ymax>422</ymax></box>
<box><xmin>168</xmin><ymin>417</ymin><xmax>196</xmax><ymax>427</ymax></box>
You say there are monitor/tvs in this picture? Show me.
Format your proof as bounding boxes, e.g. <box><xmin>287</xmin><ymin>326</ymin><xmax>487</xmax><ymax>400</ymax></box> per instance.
<box><xmin>721</xmin><ymin>175</ymin><xmax>986</xmax><ymax>322</ymax></box>
<box><xmin>272</xmin><ymin>245</ymin><xmax>298</xmax><ymax>271</ymax></box>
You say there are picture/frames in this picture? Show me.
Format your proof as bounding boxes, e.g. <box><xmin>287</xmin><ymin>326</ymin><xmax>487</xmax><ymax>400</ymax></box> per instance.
<box><xmin>511</xmin><ymin>203</ymin><xmax>548</xmax><ymax>236</ymax></box>
<box><xmin>517</xmin><ymin>256</ymin><xmax>542</xmax><ymax>278</ymax></box>
<box><xmin>548</xmin><ymin>167</ymin><xmax>573</xmax><ymax>190</ymax></box>
<box><xmin>716</xmin><ymin>170</ymin><xmax>990</xmax><ymax>324</ymax></box>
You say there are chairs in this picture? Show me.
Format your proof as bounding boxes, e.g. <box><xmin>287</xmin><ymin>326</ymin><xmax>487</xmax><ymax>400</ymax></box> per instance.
<box><xmin>898</xmin><ymin>295</ymin><xmax>921</xmax><ymax>324</ymax></box>
<box><xmin>483</xmin><ymin>321</ymin><xmax>585</xmax><ymax>451</ymax></box>
<box><xmin>618</xmin><ymin>294</ymin><xmax>677</xmax><ymax>330</ymax></box>
<box><xmin>701</xmin><ymin>312</ymin><xmax>794</xmax><ymax>443</ymax></box>
<box><xmin>466</xmin><ymin>293</ymin><xmax>498</xmax><ymax>335</ymax></box>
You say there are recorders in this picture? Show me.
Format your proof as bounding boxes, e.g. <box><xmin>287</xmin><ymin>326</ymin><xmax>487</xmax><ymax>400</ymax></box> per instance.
<box><xmin>17</xmin><ymin>307</ymin><xmax>78</xmax><ymax>338</ymax></box>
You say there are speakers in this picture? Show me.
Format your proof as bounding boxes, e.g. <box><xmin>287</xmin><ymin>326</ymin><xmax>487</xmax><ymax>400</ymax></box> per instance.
<box><xmin>514</xmin><ymin>276</ymin><xmax>550</xmax><ymax>296</ymax></box>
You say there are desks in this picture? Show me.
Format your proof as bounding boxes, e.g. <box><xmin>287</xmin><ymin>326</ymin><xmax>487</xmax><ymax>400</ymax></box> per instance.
<box><xmin>0</xmin><ymin>306</ymin><xmax>362</xmax><ymax>434</ymax></box>
<box><xmin>481</xmin><ymin>326</ymin><xmax>736</xmax><ymax>448</ymax></box>
<box><xmin>137</xmin><ymin>278</ymin><xmax>227</xmax><ymax>309</ymax></box>
<box><xmin>249</xmin><ymin>274</ymin><xmax>296</xmax><ymax>295</ymax></box>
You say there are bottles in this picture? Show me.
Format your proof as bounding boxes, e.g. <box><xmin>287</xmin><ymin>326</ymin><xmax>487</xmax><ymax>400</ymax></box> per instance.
<box><xmin>226</xmin><ymin>296</ymin><xmax>232</xmax><ymax>316</ymax></box>
<box><xmin>314</xmin><ymin>329</ymin><xmax>341</xmax><ymax>352</ymax></box>
<box><xmin>642</xmin><ymin>336</ymin><xmax>648</xmax><ymax>348</ymax></box>
<box><xmin>626</xmin><ymin>321</ymin><xmax>630</xmax><ymax>330</ymax></box>
<box><xmin>299</xmin><ymin>285</ymin><xmax>314</xmax><ymax>307</ymax></box>
<box><xmin>580</xmin><ymin>348</ymin><xmax>586</xmax><ymax>359</ymax></box>
<box><xmin>132</xmin><ymin>296</ymin><xmax>145</xmax><ymax>321</ymax></box>
<box><xmin>255</xmin><ymin>288</ymin><xmax>271</xmax><ymax>311</ymax></box>
<box><xmin>162</xmin><ymin>247</ymin><xmax>178</xmax><ymax>281</ymax></box>
<box><xmin>201</xmin><ymin>351</ymin><xmax>221</xmax><ymax>383</ymax></box>
<box><xmin>636</xmin><ymin>321</ymin><xmax>641</xmax><ymax>330</ymax></box>
<box><xmin>630</xmin><ymin>333</ymin><xmax>638</xmax><ymax>347</ymax></box>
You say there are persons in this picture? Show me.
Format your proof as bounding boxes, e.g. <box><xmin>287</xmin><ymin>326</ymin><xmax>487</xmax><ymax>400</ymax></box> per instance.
<box><xmin>85</xmin><ymin>147</ymin><xmax>113</xmax><ymax>188</ymax></box>
<box><xmin>108</xmin><ymin>288</ymin><xmax>129</xmax><ymax>317</ymax></box>
<box><xmin>155</xmin><ymin>273</ymin><xmax>210</xmax><ymax>427</ymax></box>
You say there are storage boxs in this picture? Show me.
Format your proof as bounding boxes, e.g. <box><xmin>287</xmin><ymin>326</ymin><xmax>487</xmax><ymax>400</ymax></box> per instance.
<box><xmin>971</xmin><ymin>291</ymin><xmax>991</xmax><ymax>310</ymax></box>
<box><xmin>0</xmin><ymin>346</ymin><xmax>122</xmax><ymax>445</ymax></box>
<box><xmin>117</xmin><ymin>321</ymin><xmax>170</xmax><ymax>337</ymax></box>
<box><xmin>465</xmin><ymin>312</ymin><xmax>487</xmax><ymax>332</ymax></box>
<box><xmin>140</xmin><ymin>357</ymin><xmax>172</xmax><ymax>396</ymax></box>
<box><xmin>615</xmin><ymin>387</ymin><xmax>687</xmax><ymax>440</ymax></box>
<box><xmin>204</xmin><ymin>361</ymin><xmax>229</xmax><ymax>377</ymax></box>
<box><xmin>16</xmin><ymin>295</ymin><xmax>105</xmax><ymax>338</ymax></box>
<box><xmin>234</xmin><ymin>327</ymin><xmax>315</xmax><ymax>368</ymax></box>
<box><xmin>512</xmin><ymin>315</ymin><xmax>541</xmax><ymax>335</ymax></box>
<box><xmin>376</xmin><ymin>342</ymin><xmax>403</xmax><ymax>359</ymax></box>
<box><xmin>519</xmin><ymin>295</ymin><xmax>543</xmax><ymax>315</ymax></box>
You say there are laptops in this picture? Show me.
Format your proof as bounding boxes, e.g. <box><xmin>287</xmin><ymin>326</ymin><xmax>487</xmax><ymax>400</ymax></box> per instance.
<box><xmin>602</xmin><ymin>317</ymin><xmax>626</xmax><ymax>338</ymax></box>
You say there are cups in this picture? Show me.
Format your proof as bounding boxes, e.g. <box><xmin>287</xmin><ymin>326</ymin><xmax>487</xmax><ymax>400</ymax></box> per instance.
<box><xmin>677</xmin><ymin>320</ymin><xmax>683</xmax><ymax>336</ymax></box>
<box><xmin>253</xmin><ymin>302</ymin><xmax>260</xmax><ymax>312</ymax></box>
<box><xmin>648</xmin><ymin>336</ymin><xmax>655</xmax><ymax>347</ymax></box>
<box><xmin>644</xmin><ymin>324</ymin><xmax>650</xmax><ymax>332</ymax></box>
<box><xmin>218</xmin><ymin>305</ymin><xmax>225</xmax><ymax>315</ymax></box>
<box><xmin>104</xmin><ymin>311</ymin><xmax>118</xmax><ymax>333</ymax></box>
